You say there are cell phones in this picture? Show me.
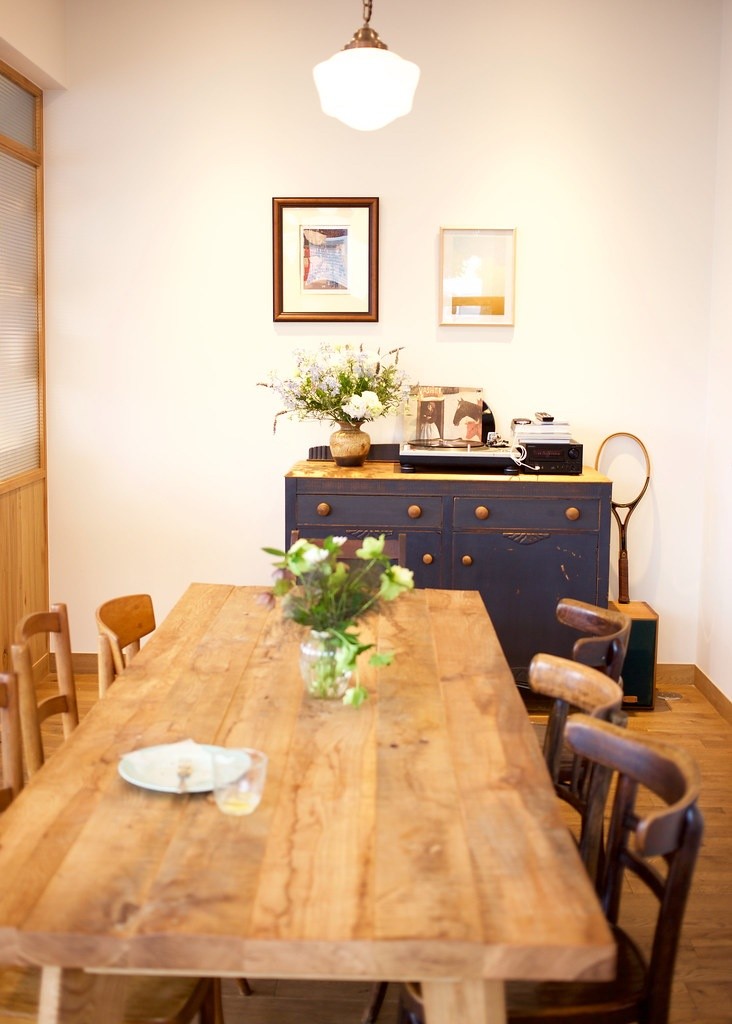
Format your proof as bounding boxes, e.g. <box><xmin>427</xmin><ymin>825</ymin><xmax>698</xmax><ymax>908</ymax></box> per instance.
<box><xmin>513</xmin><ymin>418</ymin><xmax>531</xmax><ymax>424</ymax></box>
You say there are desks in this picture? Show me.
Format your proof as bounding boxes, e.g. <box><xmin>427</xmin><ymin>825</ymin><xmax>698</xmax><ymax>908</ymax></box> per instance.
<box><xmin>1</xmin><ymin>582</ymin><xmax>615</xmax><ymax>1024</ymax></box>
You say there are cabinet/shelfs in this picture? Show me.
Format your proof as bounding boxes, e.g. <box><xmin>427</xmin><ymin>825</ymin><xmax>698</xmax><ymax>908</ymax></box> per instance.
<box><xmin>285</xmin><ymin>460</ymin><xmax>613</xmax><ymax>714</ymax></box>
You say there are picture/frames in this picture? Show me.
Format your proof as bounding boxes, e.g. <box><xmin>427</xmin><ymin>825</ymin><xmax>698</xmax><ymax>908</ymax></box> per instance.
<box><xmin>438</xmin><ymin>225</ymin><xmax>517</xmax><ymax>328</ymax></box>
<box><xmin>272</xmin><ymin>197</ymin><xmax>380</xmax><ymax>322</ymax></box>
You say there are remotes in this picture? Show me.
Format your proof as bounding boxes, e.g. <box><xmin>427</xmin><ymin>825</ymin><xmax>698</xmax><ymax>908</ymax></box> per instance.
<box><xmin>535</xmin><ymin>412</ymin><xmax>554</xmax><ymax>422</ymax></box>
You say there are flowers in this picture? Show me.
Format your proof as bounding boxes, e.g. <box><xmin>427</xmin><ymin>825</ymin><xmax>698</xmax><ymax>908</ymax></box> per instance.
<box><xmin>254</xmin><ymin>536</ymin><xmax>414</xmax><ymax>710</ymax></box>
<box><xmin>257</xmin><ymin>340</ymin><xmax>420</xmax><ymax>436</ymax></box>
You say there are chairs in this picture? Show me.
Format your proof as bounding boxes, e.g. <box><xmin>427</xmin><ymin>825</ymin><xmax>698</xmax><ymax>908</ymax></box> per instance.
<box><xmin>287</xmin><ymin>530</ymin><xmax>406</xmax><ymax>585</ymax></box>
<box><xmin>0</xmin><ymin>595</ymin><xmax>252</xmax><ymax>1024</ymax></box>
<box><xmin>361</xmin><ymin>598</ymin><xmax>712</xmax><ymax>1024</ymax></box>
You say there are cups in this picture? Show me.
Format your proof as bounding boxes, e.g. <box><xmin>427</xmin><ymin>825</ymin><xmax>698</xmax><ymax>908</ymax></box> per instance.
<box><xmin>208</xmin><ymin>749</ymin><xmax>268</xmax><ymax>815</ymax></box>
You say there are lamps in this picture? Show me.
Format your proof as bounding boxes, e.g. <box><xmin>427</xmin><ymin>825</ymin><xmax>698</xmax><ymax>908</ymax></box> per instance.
<box><xmin>310</xmin><ymin>1</ymin><xmax>422</xmax><ymax>131</ymax></box>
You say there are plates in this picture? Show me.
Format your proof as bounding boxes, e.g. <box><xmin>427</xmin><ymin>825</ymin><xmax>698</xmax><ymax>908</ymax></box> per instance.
<box><xmin>118</xmin><ymin>744</ymin><xmax>250</xmax><ymax>794</ymax></box>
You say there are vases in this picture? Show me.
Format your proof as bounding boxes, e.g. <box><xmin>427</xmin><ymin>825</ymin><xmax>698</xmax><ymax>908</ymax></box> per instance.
<box><xmin>299</xmin><ymin>630</ymin><xmax>357</xmax><ymax>699</ymax></box>
<box><xmin>330</xmin><ymin>421</ymin><xmax>370</xmax><ymax>466</ymax></box>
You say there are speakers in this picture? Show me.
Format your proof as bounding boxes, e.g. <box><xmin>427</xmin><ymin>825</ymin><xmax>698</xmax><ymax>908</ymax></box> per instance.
<box><xmin>608</xmin><ymin>600</ymin><xmax>660</xmax><ymax>710</ymax></box>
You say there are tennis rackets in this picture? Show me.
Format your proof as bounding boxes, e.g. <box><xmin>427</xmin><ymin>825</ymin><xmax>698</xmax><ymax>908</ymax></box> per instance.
<box><xmin>593</xmin><ymin>431</ymin><xmax>651</xmax><ymax>605</ymax></box>
